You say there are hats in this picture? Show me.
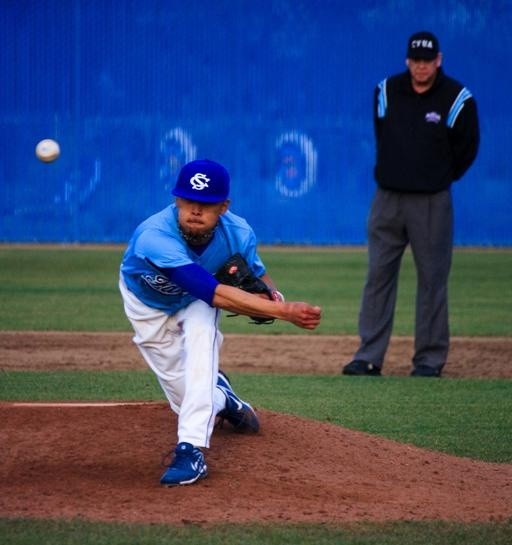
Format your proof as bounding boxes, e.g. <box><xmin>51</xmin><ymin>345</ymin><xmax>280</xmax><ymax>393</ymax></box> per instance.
<box><xmin>407</xmin><ymin>32</ymin><xmax>439</xmax><ymax>61</ymax></box>
<box><xmin>171</xmin><ymin>159</ymin><xmax>230</xmax><ymax>202</ymax></box>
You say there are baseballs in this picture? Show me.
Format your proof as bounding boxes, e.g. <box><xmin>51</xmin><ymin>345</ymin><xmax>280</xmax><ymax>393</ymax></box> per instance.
<box><xmin>36</xmin><ymin>139</ymin><xmax>59</xmax><ymax>161</ymax></box>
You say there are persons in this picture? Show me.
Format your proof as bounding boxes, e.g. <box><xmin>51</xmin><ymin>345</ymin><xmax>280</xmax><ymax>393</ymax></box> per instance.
<box><xmin>118</xmin><ymin>160</ymin><xmax>321</xmax><ymax>486</ymax></box>
<box><xmin>341</xmin><ymin>31</ymin><xmax>479</xmax><ymax>376</ymax></box>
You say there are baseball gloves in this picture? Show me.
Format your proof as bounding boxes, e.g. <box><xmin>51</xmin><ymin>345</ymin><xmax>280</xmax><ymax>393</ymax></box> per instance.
<box><xmin>214</xmin><ymin>253</ymin><xmax>284</xmax><ymax>325</ymax></box>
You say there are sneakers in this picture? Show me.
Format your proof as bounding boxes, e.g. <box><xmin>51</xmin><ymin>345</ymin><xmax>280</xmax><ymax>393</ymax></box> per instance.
<box><xmin>411</xmin><ymin>365</ymin><xmax>442</xmax><ymax>377</ymax></box>
<box><xmin>343</xmin><ymin>359</ymin><xmax>382</xmax><ymax>376</ymax></box>
<box><xmin>214</xmin><ymin>370</ymin><xmax>259</xmax><ymax>435</ymax></box>
<box><xmin>159</xmin><ymin>442</ymin><xmax>208</xmax><ymax>488</ymax></box>
